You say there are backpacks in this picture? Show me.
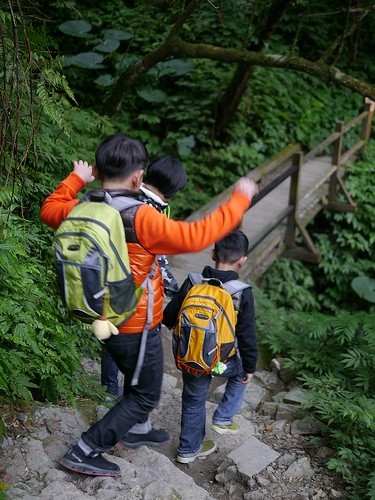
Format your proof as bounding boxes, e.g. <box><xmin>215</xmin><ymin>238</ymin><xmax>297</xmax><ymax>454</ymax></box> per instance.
<box><xmin>52</xmin><ymin>187</ymin><xmax>156</xmax><ymax>386</ymax></box>
<box><xmin>172</xmin><ymin>272</ymin><xmax>252</xmax><ymax>377</ymax></box>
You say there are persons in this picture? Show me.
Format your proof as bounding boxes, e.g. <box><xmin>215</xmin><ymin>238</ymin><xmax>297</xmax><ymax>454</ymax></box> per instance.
<box><xmin>162</xmin><ymin>230</ymin><xmax>259</xmax><ymax>464</ymax></box>
<box><xmin>99</xmin><ymin>157</ymin><xmax>186</xmax><ymax>403</ymax></box>
<box><xmin>39</xmin><ymin>132</ymin><xmax>259</xmax><ymax>476</ymax></box>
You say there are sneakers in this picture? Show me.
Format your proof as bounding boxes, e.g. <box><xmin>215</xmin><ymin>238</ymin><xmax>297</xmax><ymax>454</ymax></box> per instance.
<box><xmin>121</xmin><ymin>429</ymin><xmax>170</xmax><ymax>448</ymax></box>
<box><xmin>177</xmin><ymin>439</ymin><xmax>217</xmax><ymax>463</ymax></box>
<box><xmin>57</xmin><ymin>444</ymin><xmax>121</xmax><ymax>476</ymax></box>
<box><xmin>209</xmin><ymin>420</ymin><xmax>238</xmax><ymax>435</ymax></box>
